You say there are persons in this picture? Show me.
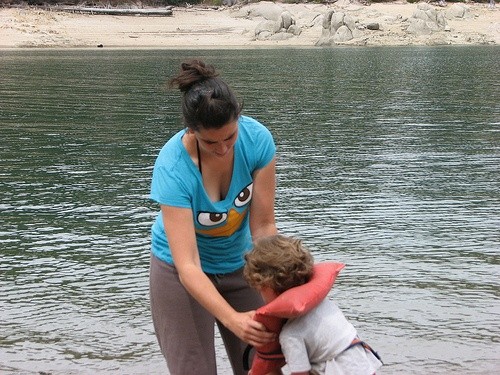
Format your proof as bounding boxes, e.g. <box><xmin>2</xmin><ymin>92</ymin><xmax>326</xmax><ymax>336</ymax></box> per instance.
<box><xmin>240</xmin><ymin>234</ymin><xmax>385</xmax><ymax>375</ymax></box>
<box><xmin>147</xmin><ymin>59</ymin><xmax>279</xmax><ymax>374</ymax></box>
